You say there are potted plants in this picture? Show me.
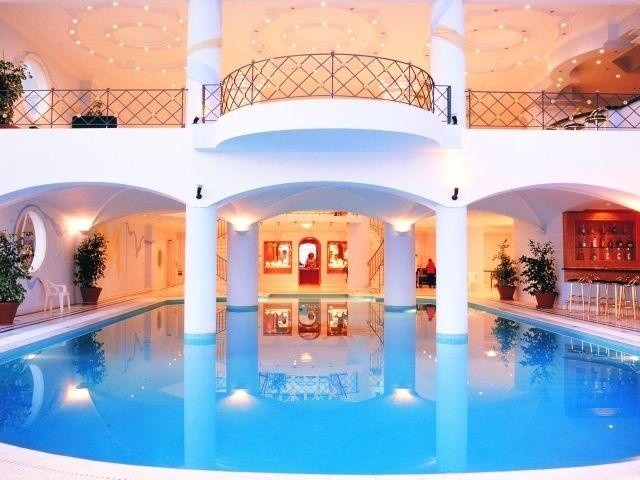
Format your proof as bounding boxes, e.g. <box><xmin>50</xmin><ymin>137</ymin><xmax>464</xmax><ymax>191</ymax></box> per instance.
<box><xmin>71</xmin><ymin>231</ymin><xmax>110</xmax><ymax>304</ymax></box>
<box><xmin>0</xmin><ymin>230</ymin><xmax>35</xmax><ymax>327</ymax></box>
<box><xmin>488</xmin><ymin>238</ymin><xmax>559</xmax><ymax>309</ymax></box>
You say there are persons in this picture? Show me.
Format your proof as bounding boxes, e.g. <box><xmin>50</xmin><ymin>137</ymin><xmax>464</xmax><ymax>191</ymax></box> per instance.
<box><xmin>281</xmin><ymin>250</ymin><xmax>287</xmax><ymax>263</ymax></box>
<box><xmin>305</xmin><ymin>253</ymin><xmax>316</xmax><ymax>267</ymax></box>
<box><xmin>425</xmin><ymin>258</ymin><xmax>436</xmax><ymax>288</ymax></box>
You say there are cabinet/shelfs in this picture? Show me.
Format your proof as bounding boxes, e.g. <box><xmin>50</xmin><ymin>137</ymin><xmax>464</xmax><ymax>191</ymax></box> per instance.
<box><xmin>563</xmin><ymin>208</ymin><xmax>640</xmax><ymax>270</ymax></box>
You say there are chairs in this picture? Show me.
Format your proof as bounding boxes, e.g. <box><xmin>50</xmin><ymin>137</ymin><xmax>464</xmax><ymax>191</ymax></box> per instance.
<box><xmin>563</xmin><ymin>106</ymin><xmax>609</xmax><ymax>130</ymax></box>
<box><xmin>415</xmin><ymin>268</ymin><xmax>434</xmax><ymax>289</ymax></box>
<box><xmin>40</xmin><ymin>278</ymin><xmax>71</xmax><ymax>312</ymax></box>
<box><xmin>566</xmin><ymin>274</ymin><xmax>640</xmax><ymax>320</ymax></box>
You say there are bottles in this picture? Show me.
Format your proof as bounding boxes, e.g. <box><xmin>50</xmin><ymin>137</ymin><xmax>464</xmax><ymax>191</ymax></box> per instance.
<box><xmin>579</xmin><ymin>223</ymin><xmax>634</xmax><ymax>261</ymax></box>
